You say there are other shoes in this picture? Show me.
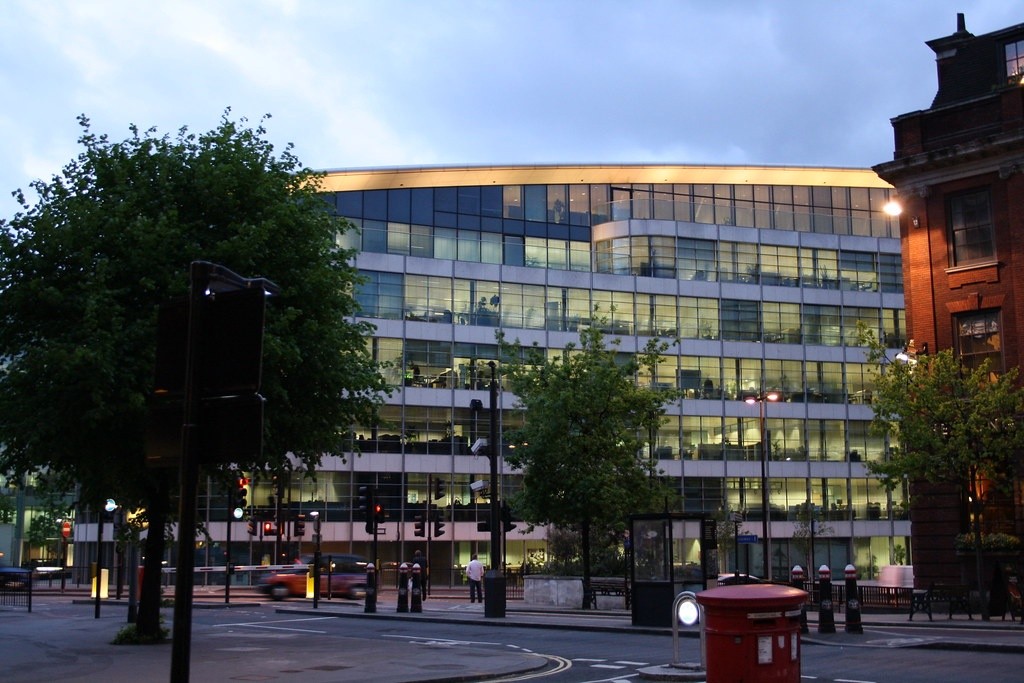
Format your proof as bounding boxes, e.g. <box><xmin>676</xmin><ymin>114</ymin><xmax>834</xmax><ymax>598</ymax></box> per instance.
<box><xmin>478</xmin><ymin>598</ymin><xmax>482</xmax><ymax>603</ymax></box>
<box><xmin>471</xmin><ymin>599</ymin><xmax>475</xmax><ymax>603</ymax></box>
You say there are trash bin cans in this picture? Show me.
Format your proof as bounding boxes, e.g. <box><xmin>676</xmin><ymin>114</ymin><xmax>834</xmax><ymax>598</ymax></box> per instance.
<box><xmin>485</xmin><ymin>570</ymin><xmax>506</xmax><ymax>617</ymax></box>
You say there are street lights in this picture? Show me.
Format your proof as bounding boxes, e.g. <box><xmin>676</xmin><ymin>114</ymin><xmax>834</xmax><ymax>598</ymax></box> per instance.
<box><xmin>469</xmin><ymin>399</ymin><xmax>484</xmax><ymax>561</ymax></box>
<box><xmin>745</xmin><ymin>393</ymin><xmax>778</xmax><ymax>579</ymax></box>
<box><xmin>488</xmin><ymin>360</ymin><xmax>498</xmax><ymax>570</ymax></box>
<box><xmin>310</xmin><ymin>512</ymin><xmax>322</xmax><ymax>608</ymax></box>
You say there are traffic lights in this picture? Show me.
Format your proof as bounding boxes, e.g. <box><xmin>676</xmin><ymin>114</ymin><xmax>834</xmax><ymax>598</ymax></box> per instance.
<box><xmin>414</xmin><ymin>501</ymin><xmax>426</xmax><ymax>537</ymax></box>
<box><xmin>294</xmin><ymin>513</ymin><xmax>306</xmax><ymax>536</ymax></box>
<box><xmin>504</xmin><ymin>504</ymin><xmax>517</xmax><ymax>532</ymax></box>
<box><xmin>234</xmin><ymin>476</ymin><xmax>249</xmax><ymax>520</ymax></box>
<box><xmin>434</xmin><ymin>509</ymin><xmax>445</xmax><ymax>538</ymax></box>
<box><xmin>477</xmin><ymin>505</ymin><xmax>491</xmax><ymax>532</ymax></box>
<box><xmin>434</xmin><ymin>477</ymin><xmax>446</xmax><ymax>499</ymax></box>
<box><xmin>264</xmin><ymin>522</ymin><xmax>276</xmax><ymax>535</ymax></box>
<box><xmin>376</xmin><ymin>504</ymin><xmax>385</xmax><ymax>523</ymax></box>
<box><xmin>360</xmin><ymin>503</ymin><xmax>374</xmax><ymax>535</ymax></box>
<box><xmin>248</xmin><ymin>517</ymin><xmax>257</xmax><ymax>536</ymax></box>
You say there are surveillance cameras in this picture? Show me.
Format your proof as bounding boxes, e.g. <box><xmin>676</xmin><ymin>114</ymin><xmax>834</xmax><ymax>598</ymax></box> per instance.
<box><xmin>470</xmin><ymin>480</ymin><xmax>489</xmax><ymax>490</ymax></box>
<box><xmin>471</xmin><ymin>439</ymin><xmax>488</xmax><ymax>454</ymax></box>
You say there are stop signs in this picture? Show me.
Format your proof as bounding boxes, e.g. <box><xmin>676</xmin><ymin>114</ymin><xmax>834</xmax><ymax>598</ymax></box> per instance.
<box><xmin>62</xmin><ymin>522</ymin><xmax>70</xmax><ymax>538</ymax></box>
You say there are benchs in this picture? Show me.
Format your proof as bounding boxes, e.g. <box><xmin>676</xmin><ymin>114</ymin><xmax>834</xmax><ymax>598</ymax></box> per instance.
<box><xmin>580</xmin><ymin>577</ymin><xmax>631</xmax><ymax>610</ymax></box>
<box><xmin>908</xmin><ymin>576</ymin><xmax>975</xmax><ymax>624</ymax></box>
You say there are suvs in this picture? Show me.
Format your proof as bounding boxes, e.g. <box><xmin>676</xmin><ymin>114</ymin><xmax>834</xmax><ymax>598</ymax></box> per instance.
<box><xmin>258</xmin><ymin>553</ymin><xmax>378</xmax><ymax>599</ymax></box>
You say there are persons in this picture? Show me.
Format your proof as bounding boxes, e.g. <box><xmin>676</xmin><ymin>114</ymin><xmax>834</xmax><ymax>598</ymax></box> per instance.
<box><xmin>466</xmin><ymin>554</ymin><xmax>484</xmax><ymax>603</ymax></box>
<box><xmin>413</xmin><ymin>550</ymin><xmax>427</xmax><ymax>601</ymax></box>
